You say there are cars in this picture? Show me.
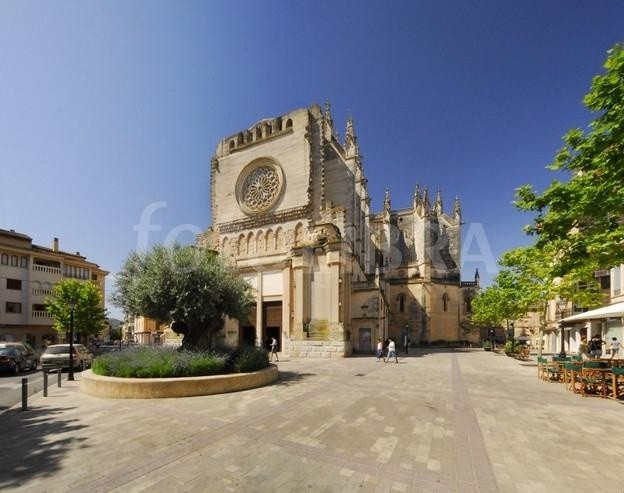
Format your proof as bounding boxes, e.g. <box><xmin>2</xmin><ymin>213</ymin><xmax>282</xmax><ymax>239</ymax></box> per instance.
<box><xmin>104</xmin><ymin>339</ymin><xmax>138</xmax><ymax>345</ymax></box>
<box><xmin>0</xmin><ymin>342</ymin><xmax>94</xmax><ymax>375</ymax></box>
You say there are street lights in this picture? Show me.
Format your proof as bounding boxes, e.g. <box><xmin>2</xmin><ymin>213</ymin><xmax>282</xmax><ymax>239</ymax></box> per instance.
<box><xmin>556</xmin><ymin>299</ymin><xmax>568</xmax><ymax>358</ymax></box>
<box><xmin>405</xmin><ymin>323</ymin><xmax>408</xmax><ymax>354</ymax></box>
<box><xmin>510</xmin><ymin>322</ymin><xmax>514</xmax><ymax>353</ymax></box>
<box><xmin>490</xmin><ymin>329</ymin><xmax>493</xmax><ymax>350</ymax></box>
<box><xmin>118</xmin><ymin>323</ymin><xmax>123</xmax><ymax>352</ymax></box>
<box><xmin>66</xmin><ymin>302</ymin><xmax>76</xmax><ymax>381</ymax></box>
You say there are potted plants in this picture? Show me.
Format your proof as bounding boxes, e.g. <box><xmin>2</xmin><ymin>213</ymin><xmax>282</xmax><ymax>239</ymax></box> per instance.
<box><xmin>483</xmin><ymin>339</ymin><xmax>491</xmax><ymax>351</ymax></box>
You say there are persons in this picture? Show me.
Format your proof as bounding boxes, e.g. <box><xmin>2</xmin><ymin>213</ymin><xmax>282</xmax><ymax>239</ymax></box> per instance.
<box><xmin>373</xmin><ymin>337</ymin><xmax>385</xmax><ymax>361</ymax></box>
<box><xmin>590</xmin><ymin>333</ymin><xmax>606</xmax><ymax>358</ymax></box>
<box><xmin>586</xmin><ymin>336</ymin><xmax>595</xmax><ymax>354</ymax></box>
<box><xmin>577</xmin><ymin>334</ymin><xmax>588</xmax><ymax>356</ymax></box>
<box><xmin>384</xmin><ymin>336</ymin><xmax>398</xmax><ymax>362</ymax></box>
<box><xmin>267</xmin><ymin>335</ymin><xmax>279</xmax><ymax>361</ymax></box>
<box><xmin>608</xmin><ymin>336</ymin><xmax>623</xmax><ymax>359</ymax></box>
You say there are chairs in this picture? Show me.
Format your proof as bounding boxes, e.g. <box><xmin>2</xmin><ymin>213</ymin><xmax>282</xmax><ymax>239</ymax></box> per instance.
<box><xmin>518</xmin><ymin>345</ymin><xmax>529</xmax><ymax>360</ymax></box>
<box><xmin>538</xmin><ymin>356</ymin><xmax>624</xmax><ymax>400</ymax></box>
